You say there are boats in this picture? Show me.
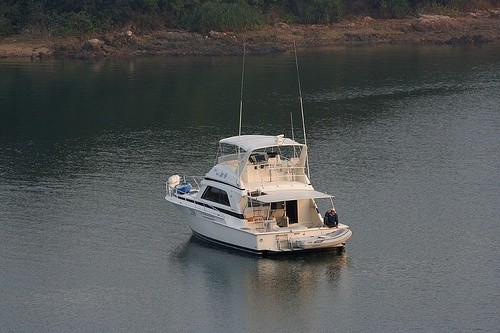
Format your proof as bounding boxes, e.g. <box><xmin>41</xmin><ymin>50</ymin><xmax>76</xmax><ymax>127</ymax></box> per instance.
<box><xmin>165</xmin><ymin>40</ymin><xmax>352</xmax><ymax>259</ymax></box>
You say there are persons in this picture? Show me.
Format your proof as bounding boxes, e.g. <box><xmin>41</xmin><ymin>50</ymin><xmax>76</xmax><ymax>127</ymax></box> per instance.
<box><xmin>324</xmin><ymin>207</ymin><xmax>339</xmax><ymax>228</ymax></box>
